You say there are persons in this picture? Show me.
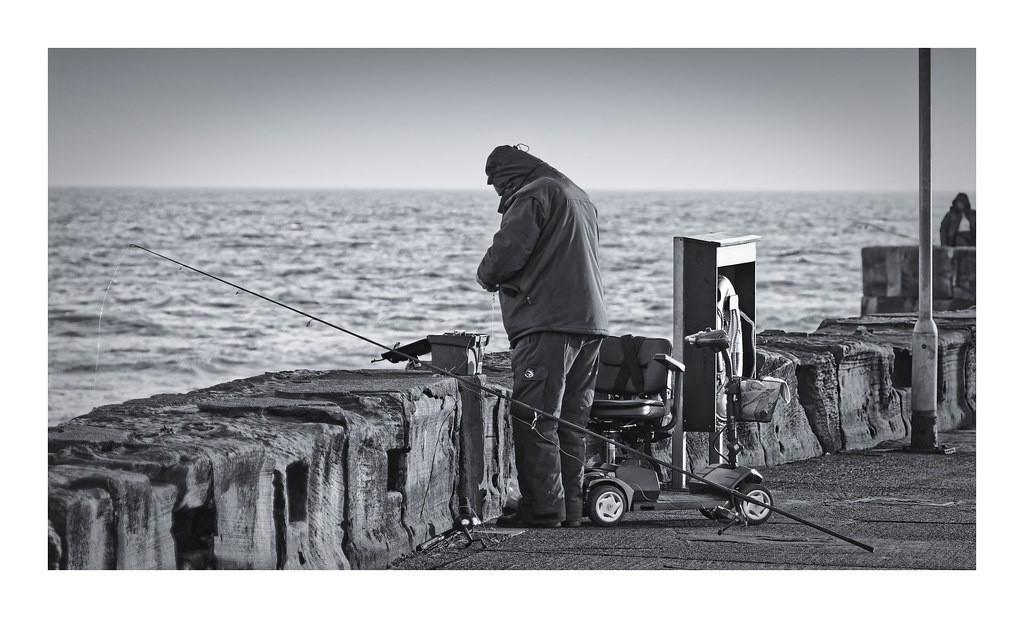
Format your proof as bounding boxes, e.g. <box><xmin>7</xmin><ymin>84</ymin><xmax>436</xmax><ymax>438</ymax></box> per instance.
<box><xmin>940</xmin><ymin>192</ymin><xmax>976</xmax><ymax>247</ymax></box>
<box><xmin>477</xmin><ymin>144</ymin><xmax>610</xmax><ymax>528</ymax></box>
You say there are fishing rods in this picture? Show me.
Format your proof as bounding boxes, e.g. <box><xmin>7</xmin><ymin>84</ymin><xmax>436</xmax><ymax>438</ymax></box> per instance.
<box><xmin>120</xmin><ymin>240</ymin><xmax>877</xmax><ymax>555</ymax></box>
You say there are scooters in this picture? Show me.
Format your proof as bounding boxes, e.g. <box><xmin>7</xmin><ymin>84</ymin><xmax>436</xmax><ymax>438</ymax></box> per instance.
<box><xmin>580</xmin><ymin>329</ymin><xmax>785</xmax><ymax>529</ymax></box>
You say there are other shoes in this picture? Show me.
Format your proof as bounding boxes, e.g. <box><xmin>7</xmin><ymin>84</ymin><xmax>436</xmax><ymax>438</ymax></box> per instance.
<box><xmin>562</xmin><ymin>521</ymin><xmax>581</xmax><ymax>527</ymax></box>
<box><xmin>497</xmin><ymin>512</ymin><xmax>561</xmax><ymax>527</ymax></box>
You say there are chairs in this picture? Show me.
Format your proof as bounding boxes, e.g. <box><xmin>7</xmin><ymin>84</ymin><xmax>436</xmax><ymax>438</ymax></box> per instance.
<box><xmin>580</xmin><ymin>336</ymin><xmax>686</xmax><ymax>468</ymax></box>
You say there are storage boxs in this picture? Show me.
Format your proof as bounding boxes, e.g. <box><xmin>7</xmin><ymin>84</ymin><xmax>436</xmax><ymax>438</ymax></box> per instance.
<box><xmin>372</xmin><ymin>332</ymin><xmax>491</xmax><ymax>376</ymax></box>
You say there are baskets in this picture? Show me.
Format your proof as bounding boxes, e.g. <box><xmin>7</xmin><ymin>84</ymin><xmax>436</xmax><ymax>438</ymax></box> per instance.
<box><xmin>735</xmin><ymin>377</ymin><xmax>781</xmax><ymax>422</ymax></box>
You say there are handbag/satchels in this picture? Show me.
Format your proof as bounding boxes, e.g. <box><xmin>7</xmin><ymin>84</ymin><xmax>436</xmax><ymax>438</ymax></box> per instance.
<box><xmin>741</xmin><ymin>376</ymin><xmax>791</xmax><ymax>420</ymax></box>
<box><xmin>596</xmin><ymin>335</ymin><xmax>672</xmax><ymax>398</ymax></box>
